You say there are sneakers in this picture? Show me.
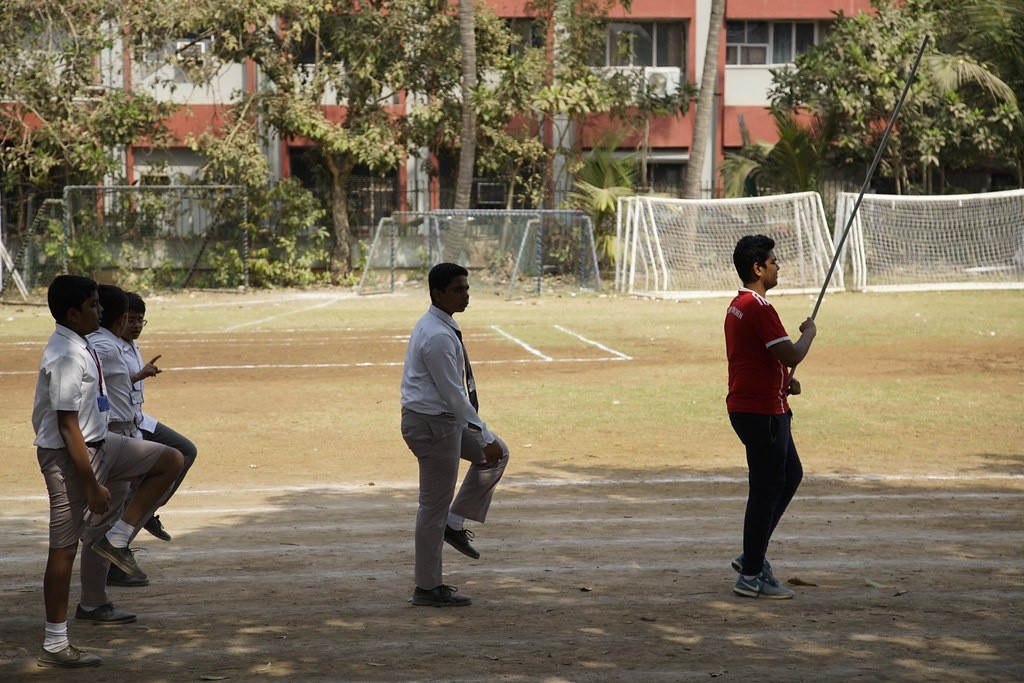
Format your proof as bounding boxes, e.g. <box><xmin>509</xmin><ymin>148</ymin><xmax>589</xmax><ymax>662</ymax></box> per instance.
<box><xmin>37</xmin><ymin>644</ymin><xmax>102</xmax><ymax>668</ymax></box>
<box><xmin>410</xmin><ymin>587</ymin><xmax>473</xmax><ymax>607</ymax></box>
<box><xmin>74</xmin><ymin>602</ymin><xmax>138</xmax><ymax>624</ymax></box>
<box><xmin>730</xmin><ymin>550</ymin><xmax>795</xmax><ymax>599</ymax></box>
<box><xmin>90</xmin><ymin>534</ymin><xmax>149</xmax><ymax>587</ymax></box>
<box><xmin>443</xmin><ymin>524</ymin><xmax>481</xmax><ymax>561</ymax></box>
<box><xmin>142</xmin><ymin>514</ymin><xmax>171</xmax><ymax>542</ymax></box>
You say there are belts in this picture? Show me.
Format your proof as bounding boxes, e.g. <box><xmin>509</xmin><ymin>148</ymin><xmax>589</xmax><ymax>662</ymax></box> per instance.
<box><xmin>85</xmin><ymin>438</ymin><xmax>105</xmax><ymax>449</ymax></box>
<box><xmin>107</xmin><ymin>421</ymin><xmax>134</xmax><ymax>430</ymax></box>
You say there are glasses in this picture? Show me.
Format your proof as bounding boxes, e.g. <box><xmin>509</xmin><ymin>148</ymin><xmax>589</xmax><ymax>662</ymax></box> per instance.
<box><xmin>126</xmin><ymin>318</ymin><xmax>147</xmax><ymax>328</ymax></box>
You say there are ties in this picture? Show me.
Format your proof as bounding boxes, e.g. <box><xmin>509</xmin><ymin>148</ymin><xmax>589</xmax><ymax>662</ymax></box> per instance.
<box><xmin>453</xmin><ymin>328</ymin><xmax>479</xmax><ymax>413</ymax></box>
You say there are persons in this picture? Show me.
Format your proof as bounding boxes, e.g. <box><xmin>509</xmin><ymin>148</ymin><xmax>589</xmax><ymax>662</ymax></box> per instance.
<box><xmin>400</xmin><ymin>262</ymin><xmax>510</xmax><ymax>608</ymax></box>
<box><xmin>724</xmin><ymin>233</ymin><xmax>818</xmax><ymax>600</ymax></box>
<box><xmin>100</xmin><ymin>292</ymin><xmax>197</xmax><ymax>586</ymax></box>
<box><xmin>31</xmin><ymin>276</ymin><xmax>185</xmax><ymax>670</ymax></box>
<box><xmin>70</xmin><ymin>284</ymin><xmax>184</xmax><ymax>626</ymax></box>
